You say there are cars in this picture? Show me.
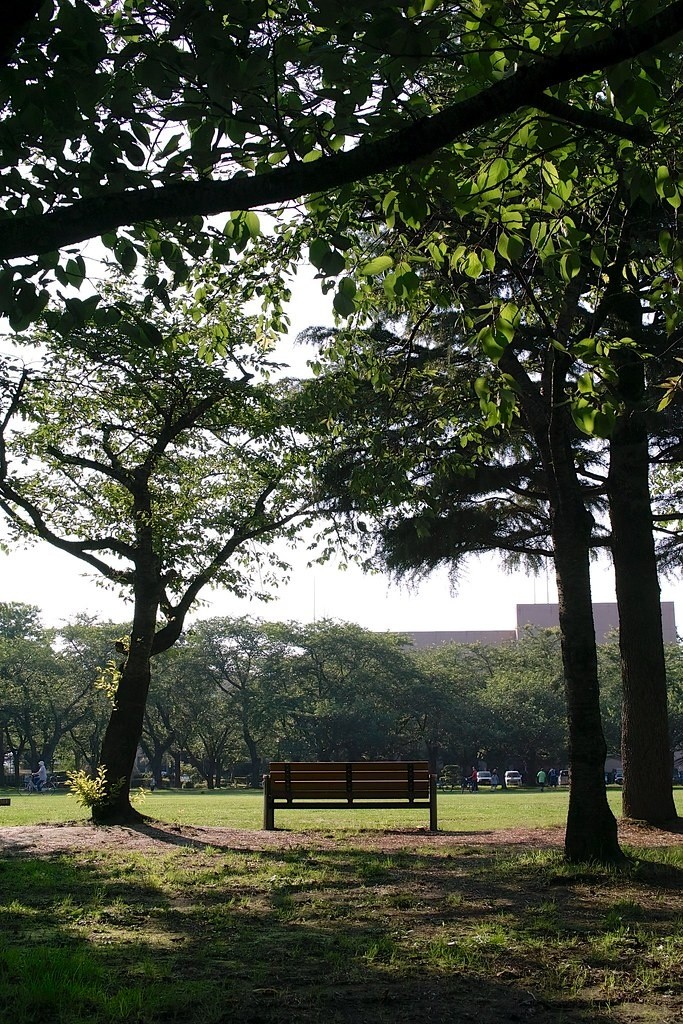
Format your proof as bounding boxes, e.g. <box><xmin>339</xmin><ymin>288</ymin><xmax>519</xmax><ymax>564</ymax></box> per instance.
<box><xmin>558</xmin><ymin>770</ymin><xmax>570</xmax><ymax>785</ymax></box>
<box><xmin>505</xmin><ymin>770</ymin><xmax>523</xmax><ymax>785</ymax></box>
<box><xmin>477</xmin><ymin>771</ymin><xmax>492</xmax><ymax>785</ymax></box>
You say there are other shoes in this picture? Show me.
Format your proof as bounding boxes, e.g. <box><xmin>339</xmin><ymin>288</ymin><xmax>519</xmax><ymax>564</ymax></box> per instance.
<box><xmin>541</xmin><ymin>789</ymin><xmax>543</xmax><ymax>792</ymax></box>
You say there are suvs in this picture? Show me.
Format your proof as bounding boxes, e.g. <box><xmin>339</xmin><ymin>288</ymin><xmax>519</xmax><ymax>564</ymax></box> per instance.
<box><xmin>615</xmin><ymin>772</ymin><xmax>624</xmax><ymax>785</ymax></box>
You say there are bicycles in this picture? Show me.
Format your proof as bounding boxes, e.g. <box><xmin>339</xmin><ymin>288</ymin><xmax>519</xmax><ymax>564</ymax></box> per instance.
<box><xmin>436</xmin><ymin>781</ymin><xmax>454</xmax><ymax>792</ymax></box>
<box><xmin>18</xmin><ymin>774</ymin><xmax>56</xmax><ymax>796</ymax></box>
<box><xmin>460</xmin><ymin>776</ymin><xmax>475</xmax><ymax>794</ymax></box>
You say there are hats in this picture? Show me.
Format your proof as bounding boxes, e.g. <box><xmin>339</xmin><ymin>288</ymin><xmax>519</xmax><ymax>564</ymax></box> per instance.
<box><xmin>38</xmin><ymin>761</ymin><xmax>44</xmax><ymax>766</ymax></box>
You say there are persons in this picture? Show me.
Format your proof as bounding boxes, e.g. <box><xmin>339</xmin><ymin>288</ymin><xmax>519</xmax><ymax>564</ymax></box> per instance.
<box><xmin>262</xmin><ymin>772</ymin><xmax>267</xmax><ymax>787</ymax></box>
<box><xmin>150</xmin><ymin>775</ymin><xmax>158</xmax><ymax>791</ymax></box>
<box><xmin>468</xmin><ymin>767</ymin><xmax>477</xmax><ymax>794</ymax></box>
<box><xmin>32</xmin><ymin>761</ymin><xmax>47</xmax><ymax>795</ymax></box>
<box><xmin>536</xmin><ymin>766</ymin><xmax>559</xmax><ymax>791</ymax></box>
<box><xmin>491</xmin><ymin>766</ymin><xmax>499</xmax><ymax>790</ymax></box>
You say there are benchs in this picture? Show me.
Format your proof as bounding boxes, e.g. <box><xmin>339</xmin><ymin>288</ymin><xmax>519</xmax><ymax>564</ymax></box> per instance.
<box><xmin>262</xmin><ymin>762</ymin><xmax>438</xmax><ymax>830</ymax></box>
